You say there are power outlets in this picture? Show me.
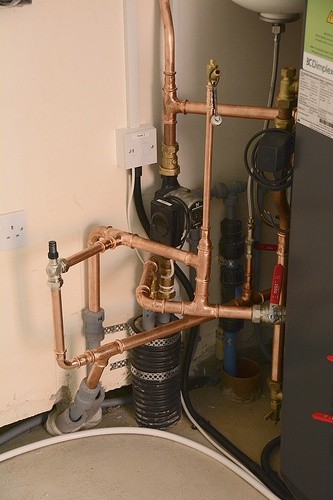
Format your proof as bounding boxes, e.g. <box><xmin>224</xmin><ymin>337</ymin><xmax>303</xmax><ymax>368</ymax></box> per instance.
<box><xmin>0</xmin><ymin>210</ymin><xmax>27</xmax><ymax>252</ymax></box>
